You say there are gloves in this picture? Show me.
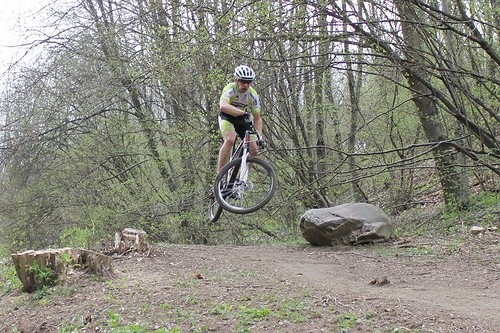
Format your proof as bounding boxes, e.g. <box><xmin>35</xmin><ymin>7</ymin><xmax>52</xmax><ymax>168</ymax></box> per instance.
<box><xmin>243</xmin><ymin>107</ymin><xmax>253</xmax><ymax>123</ymax></box>
<box><xmin>256</xmin><ymin>138</ymin><xmax>265</xmax><ymax>149</ymax></box>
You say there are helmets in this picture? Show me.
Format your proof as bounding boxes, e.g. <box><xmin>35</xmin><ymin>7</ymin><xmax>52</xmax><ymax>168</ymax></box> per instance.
<box><xmin>233</xmin><ymin>65</ymin><xmax>255</xmax><ymax>80</ymax></box>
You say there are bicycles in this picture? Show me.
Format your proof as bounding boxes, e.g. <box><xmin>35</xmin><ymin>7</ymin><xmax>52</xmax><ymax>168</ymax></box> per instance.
<box><xmin>210</xmin><ymin>109</ymin><xmax>275</xmax><ymax>224</ymax></box>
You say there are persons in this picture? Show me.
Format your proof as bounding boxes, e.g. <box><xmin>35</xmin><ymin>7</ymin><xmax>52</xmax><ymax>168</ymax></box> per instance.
<box><xmin>219</xmin><ymin>65</ymin><xmax>262</xmax><ymax>188</ymax></box>
